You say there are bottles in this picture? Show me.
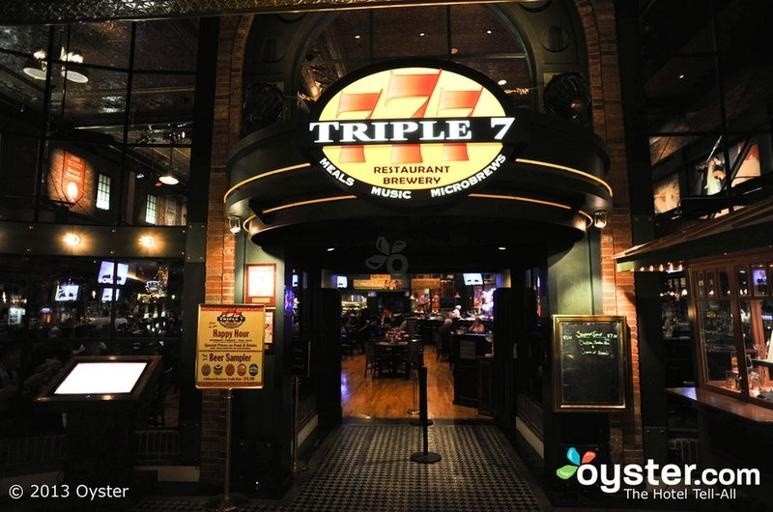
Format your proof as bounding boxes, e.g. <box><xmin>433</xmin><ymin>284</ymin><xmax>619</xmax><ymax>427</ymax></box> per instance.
<box><xmin>138</xmin><ymin>305</ymin><xmax>170</xmax><ymax>336</ymax></box>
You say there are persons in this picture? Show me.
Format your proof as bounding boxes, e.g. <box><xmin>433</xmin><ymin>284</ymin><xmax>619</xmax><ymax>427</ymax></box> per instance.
<box><xmin>341</xmin><ymin>305</ymin><xmax>484</xmax><ymax>362</ymax></box>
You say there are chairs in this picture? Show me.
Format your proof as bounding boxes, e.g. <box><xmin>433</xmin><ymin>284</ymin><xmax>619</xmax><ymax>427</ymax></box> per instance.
<box><xmin>361</xmin><ymin>324</ymin><xmax>426</xmax><ymax>377</ymax></box>
<box><xmin>435</xmin><ymin>321</ymin><xmax>491</xmax><ymax>361</ymax></box>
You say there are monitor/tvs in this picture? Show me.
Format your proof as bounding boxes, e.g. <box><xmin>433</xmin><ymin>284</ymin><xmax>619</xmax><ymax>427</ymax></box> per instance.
<box><xmin>34</xmin><ymin>354</ymin><xmax>162</xmax><ymax>403</ymax></box>
<box><xmin>55</xmin><ymin>285</ymin><xmax>79</xmax><ymax>302</ymax></box>
<box><xmin>463</xmin><ymin>273</ymin><xmax>484</xmax><ymax>286</ymax></box>
<box><xmin>101</xmin><ymin>288</ymin><xmax>120</xmax><ymax>304</ymax></box>
<box><xmin>337</xmin><ymin>275</ymin><xmax>347</xmax><ymax>288</ymax></box>
<box><xmin>97</xmin><ymin>261</ymin><xmax>129</xmax><ymax>286</ymax></box>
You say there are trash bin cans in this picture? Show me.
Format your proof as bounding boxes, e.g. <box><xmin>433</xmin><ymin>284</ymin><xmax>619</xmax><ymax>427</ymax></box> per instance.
<box><xmin>644</xmin><ymin>409</ymin><xmax>708</xmax><ymax>474</ymax></box>
